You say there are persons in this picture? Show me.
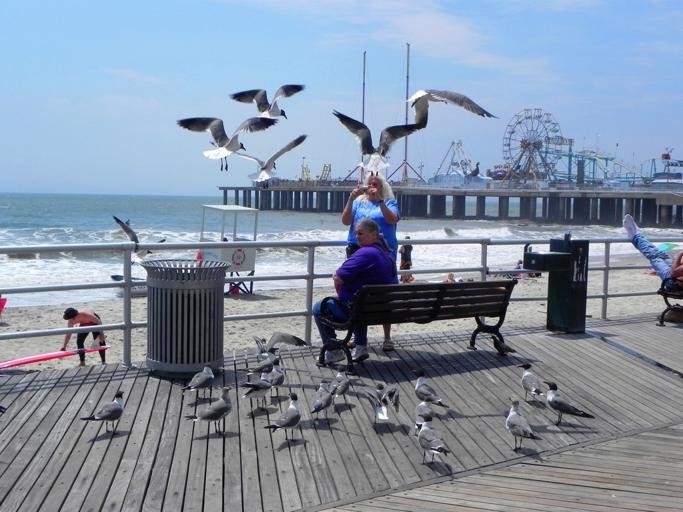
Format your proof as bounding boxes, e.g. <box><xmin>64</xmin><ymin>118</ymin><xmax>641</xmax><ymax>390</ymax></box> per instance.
<box><xmin>224</xmin><ymin>238</ymin><xmax>227</xmax><ymax>242</ymax></box>
<box><xmin>61</xmin><ymin>308</ymin><xmax>107</xmax><ymax>365</ymax></box>
<box><xmin>398</xmin><ymin>235</ymin><xmax>415</xmax><ymax>282</ymax></box>
<box><xmin>341</xmin><ymin>172</ymin><xmax>400</xmax><ymax>352</ymax></box>
<box><xmin>312</xmin><ymin>221</ymin><xmax>400</xmax><ymax>367</ymax></box>
<box><xmin>623</xmin><ymin>212</ymin><xmax>683</xmax><ymax>289</ymax></box>
<box><xmin>467</xmin><ymin>278</ymin><xmax>473</xmax><ymax>281</ymax></box>
<box><xmin>459</xmin><ymin>279</ymin><xmax>464</xmax><ymax>282</ymax></box>
<box><xmin>517</xmin><ymin>259</ymin><xmax>525</xmax><ymax>278</ymax></box>
<box><xmin>441</xmin><ymin>273</ymin><xmax>456</xmax><ymax>282</ymax></box>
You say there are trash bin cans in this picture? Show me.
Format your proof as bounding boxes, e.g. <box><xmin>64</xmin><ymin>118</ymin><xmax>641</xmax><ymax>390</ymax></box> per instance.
<box><xmin>141</xmin><ymin>259</ymin><xmax>231</xmax><ymax>379</ymax></box>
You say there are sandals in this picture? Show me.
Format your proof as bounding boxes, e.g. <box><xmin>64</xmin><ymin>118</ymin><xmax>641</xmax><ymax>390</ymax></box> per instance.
<box><xmin>383</xmin><ymin>341</ymin><xmax>394</xmax><ymax>351</ymax></box>
<box><xmin>346</xmin><ymin>338</ymin><xmax>356</xmax><ymax>348</ymax></box>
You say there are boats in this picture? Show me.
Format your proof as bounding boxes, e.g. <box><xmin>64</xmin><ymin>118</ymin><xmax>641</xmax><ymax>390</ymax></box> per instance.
<box><xmin>110</xmin><ymin>274</ymin><xmax>147</xmax><ymax>297</ymax></box>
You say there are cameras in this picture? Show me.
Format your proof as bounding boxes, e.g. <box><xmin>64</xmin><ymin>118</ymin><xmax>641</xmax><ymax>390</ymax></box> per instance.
<box><xmin>359</xmin><ymin>186</ymin><xmax>369</xmax><ymax>194</ymax></box>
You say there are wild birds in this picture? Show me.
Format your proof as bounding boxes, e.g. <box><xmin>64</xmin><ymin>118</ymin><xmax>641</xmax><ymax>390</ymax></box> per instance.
<box><xmin>331</xmin><ymin>107</ymin><xmax>424</xmax><ymax>177</ymax></box>
<box><xmin>175</xmin><ymin>115</ymin><xmax>279</xmax><ymax>173</ymax></box>
<box><xmin>404</xmin><ymin>88</ymin><xmax>501</xmax><ymax>129</ymax></box>
<box><xmin>504</xmin><ymin>396</ymin><xmax>543</xmax><ymax>453</ymax></box>
<box><xmin>80</xmin><ymin>390</ymin><xmax>125</xmax><ymax>434</ymax></box>
<box><xmin>209</xmin><ymin>133</ymin><xmax>309</xmax><ymax>190</ymax></box>
<box><xmin>516</xmin><ymin>363</ymin><xmax>544</xmax><ymax>403</ymax></box>
<box><xmin>225</xmin><ymin>83</ymin><xmax>305</xmax><ymax>121</ymax></box>
<box><xmin>543</xmin><ymin>380</ymin><xmax>596</xmax><ymax>427</ymax></box>
<box><xmin>180</xmin><ymin>330</ymin><xmax>453</xmax><ymax>466</ymax></box>
<box><xmin>112</xmin><ymin>214</ymin><xmax>167</xmax><ymax>266</ymax></box>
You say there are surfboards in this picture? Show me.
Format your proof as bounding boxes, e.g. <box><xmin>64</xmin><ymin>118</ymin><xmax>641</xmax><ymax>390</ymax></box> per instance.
<box><xmin>0</xmin><ymin>345</ymin><xmax>109</xmax><ymax>370</ymax></box>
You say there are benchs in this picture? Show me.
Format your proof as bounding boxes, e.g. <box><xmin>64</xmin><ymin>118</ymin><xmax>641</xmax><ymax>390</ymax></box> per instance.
<box><xmin>656</xmin><ymin>278</ymin><xmax>682</xmax><ymax>327</ymax></box>
<box><xmin>315</xmin><ymin>278</ymin><xmax>519</xmax><ymax>374</ymax></box>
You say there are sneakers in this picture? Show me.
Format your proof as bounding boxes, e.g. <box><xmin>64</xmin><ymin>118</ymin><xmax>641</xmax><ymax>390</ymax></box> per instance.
<box><xmin>316</xmin><ymin>350</ymin><xmax>345</xmax><ymax>364</ymax></box>
<box><xmin>351</xmin><ymin>345</ymin><xmax>369</xmax><ymax>361</ymax></box>
